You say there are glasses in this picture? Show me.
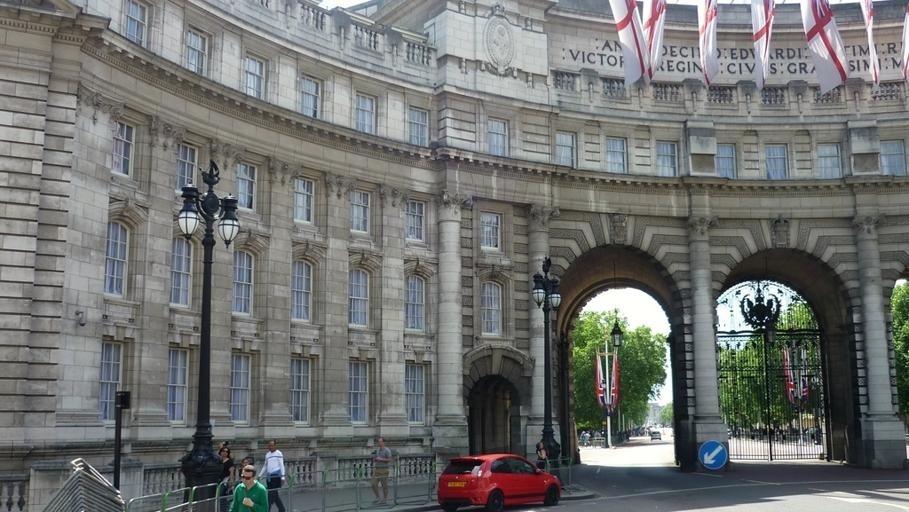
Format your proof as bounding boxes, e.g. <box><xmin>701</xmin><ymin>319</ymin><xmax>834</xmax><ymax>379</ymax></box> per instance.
<box><xmin>241</xmin><ymin>475</ymin><xmax>253</xmax><ymax>480</ymax></box>
<box><xmin>222</xmin><ymin>450</ymin><xmax>229</xmax><ymax>453</ymax></box>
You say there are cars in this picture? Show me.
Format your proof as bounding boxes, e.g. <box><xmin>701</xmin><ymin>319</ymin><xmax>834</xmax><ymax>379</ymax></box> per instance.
<box><xmin>435</xmin><ymin>451</ymin><xmax>561</xmax><ymax>510</ymax></box>
<box><xmin>651</xmin><ymin>431</ymin><xmax>661</xmax><ymax>440</ymax></box>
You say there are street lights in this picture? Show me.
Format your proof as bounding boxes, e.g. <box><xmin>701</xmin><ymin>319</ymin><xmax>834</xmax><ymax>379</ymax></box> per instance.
<box><xmin>531</xmin><ymin>255</ymin><xmax>564</xmax><ymax>463</ymax></box>
<box><xmin>611</xmin><ymin>310</ymin><xmax>624</xmax><ymax>445</ymax></box>
<box><xmin>172</xmin><ymin>157</ymin><xmax>241</xmax><ymax>503</ymax></box>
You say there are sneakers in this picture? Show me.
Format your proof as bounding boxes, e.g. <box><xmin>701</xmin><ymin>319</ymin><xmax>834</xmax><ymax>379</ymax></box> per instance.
<box><xmin>378</xmin><ymin>500</ymin><xmax>387</xmax><ymax>505</ymax></box>
<box><xmin>373</xmin><ymin>496</ymin><xmax>382</xmax><ymax>504</ymax></box>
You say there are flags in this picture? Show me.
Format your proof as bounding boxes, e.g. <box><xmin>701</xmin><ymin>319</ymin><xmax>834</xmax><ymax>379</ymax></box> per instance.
<box><xmin>799</xmin><ymin>0</ymin><xmax>849</xmax><ymax>97</ymax></box>
<box><xmin>751</xmin><ymin>0</ymin><xmax>775</xmax><ymax>90</ymax></box>
<box><xmin>696</xmin><ymin>0</ymin><xmax>720</xmax><ymax>91</ymax></box>
<box><xmin>901</xmin><ymin>4</ymin><xmax>909</xmax><ymax>80</ymax></box>
<box><xmin>642</xmin><ymin>0</ymin><xmax>667</xmax><ymax>86</ymax></box>
<box><xmin>608</xmin><ymin>0</ymin><xmax>650</xmax><ymax>88</ymax></box>
<box><xmin>859</xmin><ymin>0</ymin><xmax>881</xmax><ymax>86</ymax></box>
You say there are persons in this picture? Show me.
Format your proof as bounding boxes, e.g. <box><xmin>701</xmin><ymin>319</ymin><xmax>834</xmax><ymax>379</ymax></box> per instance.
<box><xmin>372</xmin><ymin>437</ymin><xmax>392</xmax><ymax>505</ymax></box>
<box><xmin>216</xmin><ymin>441</ymin><xmax>286</xmax><ymax>512</ymax></box>
<box><xmin>580</xmin><ymin>429</ymin><xmax>605</xmax><ymax>447</ymax></box>
<box><xmin>535</xmin><ymin>441</ymin><xmax>546</xmax><ymax>469</ymax></box>
